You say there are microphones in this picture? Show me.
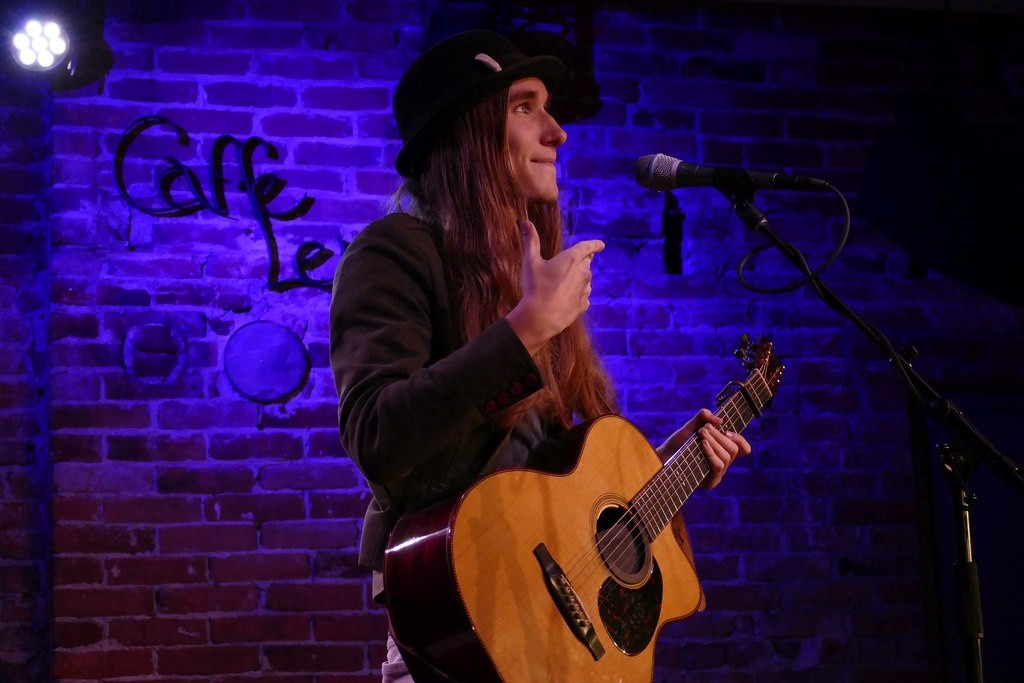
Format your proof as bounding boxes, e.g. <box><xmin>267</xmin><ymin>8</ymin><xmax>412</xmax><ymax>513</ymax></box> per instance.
<box><xmin>633</xmin><ymin>152</ymin><xmax>781</xmax><ymax>193</ymax></box>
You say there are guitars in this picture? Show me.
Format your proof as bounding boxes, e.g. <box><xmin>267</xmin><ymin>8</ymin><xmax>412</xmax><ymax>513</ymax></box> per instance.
<box><xmin>379</xmin><ymin>331</ymin><xmax>787</xmax><ymax>681</ymax></box>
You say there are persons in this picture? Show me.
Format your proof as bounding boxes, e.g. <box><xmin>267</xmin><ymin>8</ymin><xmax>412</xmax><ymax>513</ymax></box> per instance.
<box><xmin>330</xmin><ymin>34</ymin><xmax>755</xmax><ymax>683</ymax></box>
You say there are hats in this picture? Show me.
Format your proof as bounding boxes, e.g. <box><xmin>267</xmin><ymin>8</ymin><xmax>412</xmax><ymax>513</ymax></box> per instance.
<box><xmin>391</xmin><ymin>29</ymin><xmax>566</xmax><ymax>181</ymax></box>
